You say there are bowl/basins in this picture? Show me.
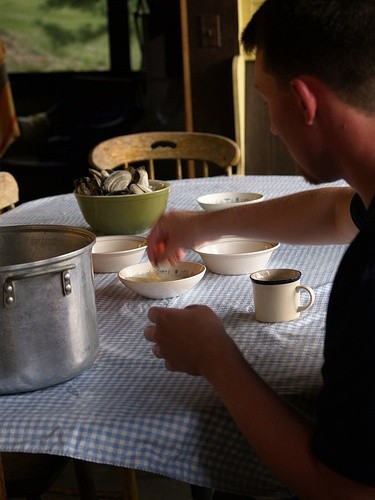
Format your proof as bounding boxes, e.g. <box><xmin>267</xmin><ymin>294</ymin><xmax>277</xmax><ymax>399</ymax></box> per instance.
<box><xmin>92</xmin><ymin>235</ymin><xmax>149</xmax><ymax>273</ymax></box>
<box><xmin>196</xmin><ymin>191</ymin><xmax>265</xmax><ymax>212</ymax></box>
<box><xmin>191</xmin><ymin>234</ymin><xmax>281</xmax><ymax>275</ymax></box>
<box><xmin>73</xmin><ymin>180</ymin><xmax>171</xmax><ymax>235</ymax></box>
<box><xmin>117</xmin><ymin>261</ymin><xmax>206</xmax><ymax>298</ymax></box>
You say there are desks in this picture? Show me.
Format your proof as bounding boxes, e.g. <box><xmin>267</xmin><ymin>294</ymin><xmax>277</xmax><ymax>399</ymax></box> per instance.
<box><xmin>0</xmin><ymin>175</ymin><xmax>351</xmax><ymax>500</ymax></box>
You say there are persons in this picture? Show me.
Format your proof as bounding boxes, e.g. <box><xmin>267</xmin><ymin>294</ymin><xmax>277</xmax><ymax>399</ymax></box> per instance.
<box><xmin>0</xmin><ymin>38</ymin><xmax>22</xmax><ymax>160</ymax></box>
<box><xmin>141</xmin><ymin>0</ymin><xmax>375</xmax><ymax>499</ymax></box>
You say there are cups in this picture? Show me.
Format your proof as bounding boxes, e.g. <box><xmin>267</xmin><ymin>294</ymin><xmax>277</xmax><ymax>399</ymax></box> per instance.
<box><xmin>249</xmin><ymin>268</ymin><xmax>316</xmax><ymax>323</ymax></box>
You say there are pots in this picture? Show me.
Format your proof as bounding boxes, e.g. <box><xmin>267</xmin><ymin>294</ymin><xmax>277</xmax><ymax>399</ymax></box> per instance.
<box><xmin>0</xmin><ymin>224</ymin><xmax>100</xmax><ymax>396</ymax></box>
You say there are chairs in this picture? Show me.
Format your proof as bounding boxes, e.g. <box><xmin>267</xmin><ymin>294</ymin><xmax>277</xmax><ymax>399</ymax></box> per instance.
<box><xmin>89</xmin><ymin>131</ymin><xmax>241</xmax><ymax>180</ymax></box>
